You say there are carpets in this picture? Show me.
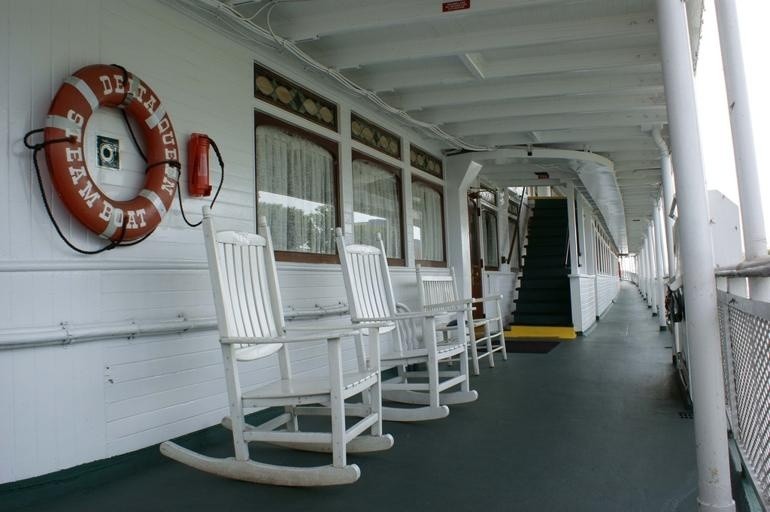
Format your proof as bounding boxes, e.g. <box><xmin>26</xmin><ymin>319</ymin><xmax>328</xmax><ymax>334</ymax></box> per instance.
<box><xmin>477</xmin><ymin>340</ymin><xmax>559</xmax><ymax>353</ymax></box>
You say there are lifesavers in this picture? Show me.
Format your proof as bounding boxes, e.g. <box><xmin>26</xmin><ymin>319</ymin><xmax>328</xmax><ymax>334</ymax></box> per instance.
<box><xmin>44</xmin><ymin>64</ymin><xmax>180</xmax><ymax>242</ymax></box>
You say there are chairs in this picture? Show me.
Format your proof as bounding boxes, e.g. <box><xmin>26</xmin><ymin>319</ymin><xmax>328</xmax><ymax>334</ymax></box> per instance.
<box><xmin>160</xmin><ymin>205</ymin><xmax>394</xmax><ymax>487</ymax></box>
<box><xmin>320</xmin><ymin>227</ymin><xmax>479</xmax><ymax>423</ymax></box>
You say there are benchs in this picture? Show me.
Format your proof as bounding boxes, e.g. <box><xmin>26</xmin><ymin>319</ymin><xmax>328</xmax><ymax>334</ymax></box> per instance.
<box><xmin>417</xmin><ymin>264</ymin><xmax>508</xmax><ymax>376</ymax></box>
